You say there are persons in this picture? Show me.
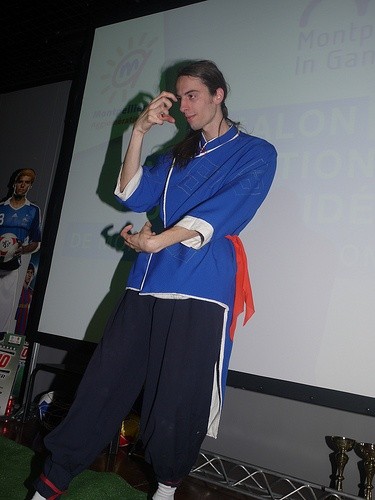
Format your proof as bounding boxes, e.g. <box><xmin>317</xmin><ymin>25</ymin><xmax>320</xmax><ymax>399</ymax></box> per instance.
<box><xmin>30</xmin><ymin>58</ymin><xmax>278</xmax><ymax>500</ymax></box>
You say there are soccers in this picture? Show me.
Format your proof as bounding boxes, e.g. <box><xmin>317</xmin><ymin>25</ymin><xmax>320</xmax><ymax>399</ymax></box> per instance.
<box><xmin>0</xmin><ymin>233</ymin><xmax>19</xmax><ymax>262</ymax></box>
